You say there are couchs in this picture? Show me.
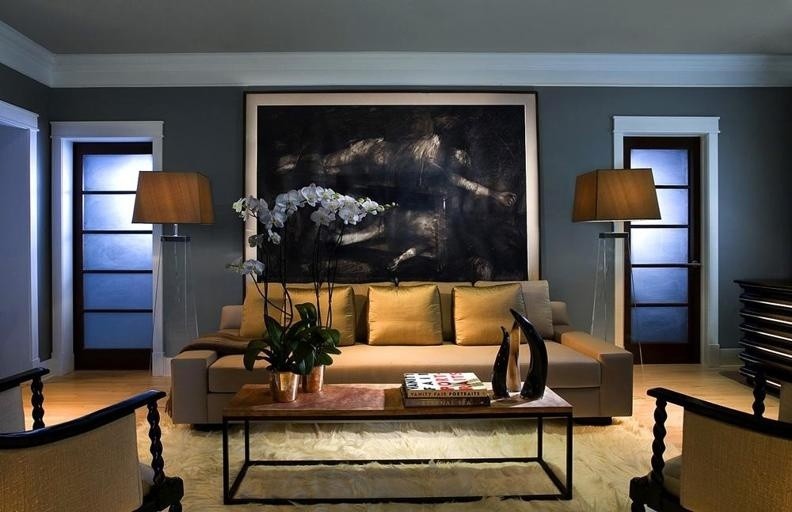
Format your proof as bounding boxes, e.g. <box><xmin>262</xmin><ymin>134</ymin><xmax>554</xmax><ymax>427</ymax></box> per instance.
<box><xmin>169</xmin><ymin>301</ymin><xmax>634</xmax><ymax>432</ymax></box>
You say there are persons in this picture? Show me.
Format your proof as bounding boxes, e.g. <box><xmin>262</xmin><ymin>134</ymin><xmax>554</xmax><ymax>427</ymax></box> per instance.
<box><xmin>259</xmin><ymin>127</ymin><xmax>518</xmax><ymax>209</ymax></box>
<box><xmin>333</xmin><ymin>204</ymin><xmax>522</xmax><ymax>281</ymax></box>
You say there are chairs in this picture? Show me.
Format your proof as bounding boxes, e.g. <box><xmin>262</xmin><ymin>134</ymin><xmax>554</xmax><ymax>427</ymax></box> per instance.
<box><xmin>0</xmin><ymin>367</ymin><xmax>182</xmax><ymax>512</ymax></box>
<box><xmin>628</xmin><ymin>367</ymin><xmax>791</xmax><ymax>509</ymax></box>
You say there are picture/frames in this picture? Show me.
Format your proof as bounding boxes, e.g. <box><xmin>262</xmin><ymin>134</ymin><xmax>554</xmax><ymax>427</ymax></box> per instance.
<box><xmin>244</xmin><ymin>90</ymin><xmax>544</xmax><ymax>283</ymax></box>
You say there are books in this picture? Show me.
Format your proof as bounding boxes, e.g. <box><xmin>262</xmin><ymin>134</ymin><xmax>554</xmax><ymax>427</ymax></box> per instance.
<box><xmin>400</xmin><ymin>387</ymin><xmax>491</xmax><ymax>408</ymax></box>
<box><xmin>403</xmin><ymin>372</ymin><xmax>488</xmax><ymax>398</ymax></box>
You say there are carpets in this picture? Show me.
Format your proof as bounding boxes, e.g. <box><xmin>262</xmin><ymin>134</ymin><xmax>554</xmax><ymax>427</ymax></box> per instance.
<box><xmin>132</xmin><ymin>406</ymin><xmax>679</xmax><ymax>512</ymax></box>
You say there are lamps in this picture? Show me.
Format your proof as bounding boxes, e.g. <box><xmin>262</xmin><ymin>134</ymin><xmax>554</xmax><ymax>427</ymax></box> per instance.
<box><xmin>132</xmin><ymin>170</ymin><xmax>216</xmax><ymax>224</ymax></box>
<box><xmin>571</xmin><ymin>167</ymin><xmax>664</xmax><ymax>224</ymax></box>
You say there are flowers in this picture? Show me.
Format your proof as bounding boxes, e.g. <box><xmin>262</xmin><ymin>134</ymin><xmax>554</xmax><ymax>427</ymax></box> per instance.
<box><xmin>224</xmin><ymin>191</ymin><xmax>315</xmax><ymax>372</ymax></box>
<box><xmin>297</xmin><ymin>185</ymin><xmax>404</xmax><ymax>366</ymax></box>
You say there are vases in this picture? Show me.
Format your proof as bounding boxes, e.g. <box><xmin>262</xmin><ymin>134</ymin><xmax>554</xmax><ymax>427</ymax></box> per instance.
<box><xmin>304</xmin><ymin>363</ymin><xmax>326</xmax><ymax>392</ymax></box>
<box><xmin>263</xmin><ymin>365</ymin><xmax>301</xmax><ymax>403</ymax></box>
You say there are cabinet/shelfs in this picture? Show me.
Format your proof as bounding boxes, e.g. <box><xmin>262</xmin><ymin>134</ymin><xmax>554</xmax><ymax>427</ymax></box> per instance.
<box><xmin>733</xmin><ymin>279</ymin><xmax>792</xmax><ymax>392</ymax></box>
